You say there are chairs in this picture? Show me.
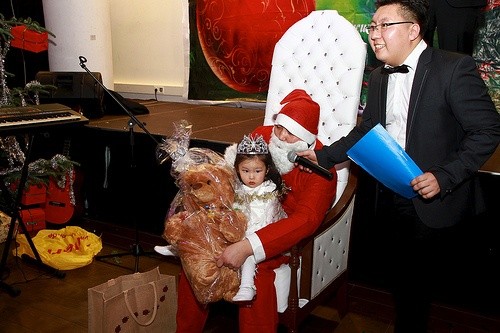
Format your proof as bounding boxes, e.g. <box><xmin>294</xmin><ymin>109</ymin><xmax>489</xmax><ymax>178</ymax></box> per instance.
<box><xmin>262</xmin><ymin>9</ymin><xmax>368</xmax><ymax>333</ymax></box>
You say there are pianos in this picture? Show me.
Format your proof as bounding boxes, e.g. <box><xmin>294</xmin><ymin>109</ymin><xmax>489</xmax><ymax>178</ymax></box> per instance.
<box><xmin>0</xmin><ymin>102</ymin><xmax>90</xmax><ymax>297</ymax></box>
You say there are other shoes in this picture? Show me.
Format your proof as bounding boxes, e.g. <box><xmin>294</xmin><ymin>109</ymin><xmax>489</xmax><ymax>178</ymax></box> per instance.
<box><xmin>232</xmin><ymin>285</ymin><xmax>256</xmax><ymax>301</ymax></box>
<box><xmin>154</xmin><ymin>245</ymin><xmax>176</xmax><ymax>256</ymax></box>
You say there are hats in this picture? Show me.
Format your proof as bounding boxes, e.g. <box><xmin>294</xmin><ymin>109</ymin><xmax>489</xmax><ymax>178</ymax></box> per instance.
<box><xmin>274</xmin><ymin>89</ymin><xmax>320</xmax><ymax>145</ymax></box>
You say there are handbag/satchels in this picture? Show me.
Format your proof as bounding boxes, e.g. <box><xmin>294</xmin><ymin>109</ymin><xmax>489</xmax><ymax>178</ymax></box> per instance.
<box><xmin>88</xmin><ymin>267</ymin><xmax>176</xmax><ymax>333</ymax></box>
<box><xmin>35</xmin><ymin>72</ymin><xmax>104</xmax><ymax>119</ymax></box>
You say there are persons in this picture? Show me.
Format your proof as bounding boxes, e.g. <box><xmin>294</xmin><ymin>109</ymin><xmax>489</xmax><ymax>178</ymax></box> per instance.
<box><xmin>294</xmin><ymin>0</ymin><xmax>500</xmax><ymax>333</ymax></box>
<box><xmin>410</xmin><ymin>0</ymin><xmax>488</xmax><ymax>57</ymax></box>
<box><xmin>155</xmin><ymin>135</ymin><xmax>288</xmax><ymax>302</ymax></box>
<box><xmin>173</xmin><ymin>89</ymin><xmax>337</xmax><ymax>333</ymax></box>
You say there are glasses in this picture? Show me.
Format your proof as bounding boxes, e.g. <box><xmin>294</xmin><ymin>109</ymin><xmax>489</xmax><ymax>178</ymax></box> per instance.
<box><xmin>365</xmin><ymin>21</ymin><xmax>414</xmax><ymax>34</ymax></box>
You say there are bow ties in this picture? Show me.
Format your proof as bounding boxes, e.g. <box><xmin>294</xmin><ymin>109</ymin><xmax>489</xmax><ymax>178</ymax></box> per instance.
<box><xmin>381</xmin><ymin>66</ymin><xmax>409</xmax><ymax>75</ymax></box>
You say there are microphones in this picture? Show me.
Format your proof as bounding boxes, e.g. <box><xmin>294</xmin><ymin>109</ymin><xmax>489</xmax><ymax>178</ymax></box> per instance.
<box><xmin>288</xmin><ymin>151</ymin><xmax>334</xmax><ymax>180</ymax></box>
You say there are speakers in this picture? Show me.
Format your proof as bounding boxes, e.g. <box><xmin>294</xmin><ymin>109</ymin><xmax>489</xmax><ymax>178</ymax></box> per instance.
<box><xmin>33</xmin><ymin>71</ymin><xmax>104</xmax><ymax>118</ymax></box>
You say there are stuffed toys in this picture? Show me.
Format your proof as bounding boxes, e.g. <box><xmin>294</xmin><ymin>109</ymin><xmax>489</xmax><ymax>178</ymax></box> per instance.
<box><xmin>164</xmin><ymin>163</ymin><xmax>247</xmax><ymax>303</ymax></box>
